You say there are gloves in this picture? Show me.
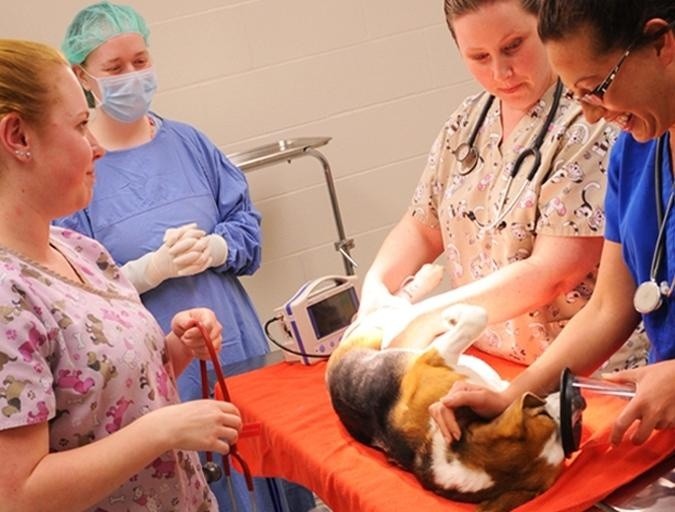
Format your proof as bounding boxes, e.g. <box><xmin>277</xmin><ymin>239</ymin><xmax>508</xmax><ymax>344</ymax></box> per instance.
<box><xmin>164</xmin><ymin>227</ymin><xmax>227</xmax><ymax>275</ymax></box>
<box><xmin>115</xmin><ymin>225</ymin><xmax>212</xmax><ymax>296</ymax></box>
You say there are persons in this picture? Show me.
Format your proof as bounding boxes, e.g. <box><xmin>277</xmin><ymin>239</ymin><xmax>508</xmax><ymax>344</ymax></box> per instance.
<box><xmin>51</xmin><ymin>2</ymin><xmax>316</xmax><ymax>511</ymax></box>
<box><xmin>356</xmin><ymin>0</ymin><xmax>655</xmax><ymax>379</ymax></box>
<box><xmin>425</xmin><ymin>0</ymin><xmax>675</xmax><ymax>448</ymax></box>
<box><xmin>0</xmin><ymin>39</ymin><xmax>243</xmax><ymax>511</ymax></box>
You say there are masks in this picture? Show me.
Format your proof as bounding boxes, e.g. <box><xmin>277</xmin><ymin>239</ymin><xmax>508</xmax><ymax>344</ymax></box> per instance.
<box><xmin>75</xmin><ymin>68</ymin><xmax>157</xmax><ymax>123</ymax></box>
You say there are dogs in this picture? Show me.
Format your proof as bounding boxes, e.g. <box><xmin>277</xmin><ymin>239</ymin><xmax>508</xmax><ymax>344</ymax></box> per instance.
<box><xmin>325</xmin><ymin>263</ymin><xmax>587</xmax><ymax>512</ymax></box>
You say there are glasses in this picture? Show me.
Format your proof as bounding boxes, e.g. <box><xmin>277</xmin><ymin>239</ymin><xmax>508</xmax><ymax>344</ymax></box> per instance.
<box><xmin>559</xmin><ymin>28</ymin><xmax>644</xmax><ymax>109</ymax></box>
